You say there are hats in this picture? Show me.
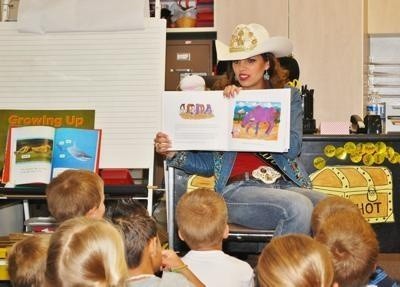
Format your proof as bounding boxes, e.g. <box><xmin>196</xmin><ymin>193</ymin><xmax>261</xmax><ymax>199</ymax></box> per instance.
<box><xmin>214</xmin><ymin>22</ymin><xmax>294</xmax><ymax>62</ymax></box>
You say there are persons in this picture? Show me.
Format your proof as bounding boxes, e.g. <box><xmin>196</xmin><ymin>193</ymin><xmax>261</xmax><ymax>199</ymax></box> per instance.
<box><xmin>154</xmin><ymin>22</ymin><xmax>326</xmax><ymax>234</ymax></box>
<box><xmin>271</xmin><ymin>37</ymin><xmax>300</xmax><ymax>83</ymax></box>
<box><xmin>6</xmin><ymin>232</ymin><xmax>54</xmax><ymax>286</ymax></box>
<box><xmin>45</xmin><ymin>214</ymin><xmax>130</xmax><ymax>286</ymax></box>
<box><xmin>311</xmin><ymin>194</ymin><xmax>400</xmax><ymax>286</ymax></box>
<box><xmin>161</xmin><ymin>187</ymin><xmax>256</xmax><ymax>286</ymax></box>
<box><xmin>103</xmin><ymin>195</ymin><xmax>206</xmax><ymax>286</ymax></box>
<box><xmin>256</xmin><ymin>234</ymin><xmax>340</xmax><ymax>286</ymax></box>
<box><xmin>314</xmin><ymin>208</ymin><xmax>377</xmax><ymax>286</ymax></box>
<box><xmin>45</xmin><ymin>168</ymin><xmax>107</xmax><ymax>220</ymax></box>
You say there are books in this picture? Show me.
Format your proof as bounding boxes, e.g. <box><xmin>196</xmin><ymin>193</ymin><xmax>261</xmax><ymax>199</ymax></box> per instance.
<box><xmin>1</xmin><ymin>123</ymin><xmax>104</xmax><ymax>184</ymax></box>
<box><xmin>159</xmin><ymin>89</ymin><xmax>292</xmax><ymax>153</ymax></box>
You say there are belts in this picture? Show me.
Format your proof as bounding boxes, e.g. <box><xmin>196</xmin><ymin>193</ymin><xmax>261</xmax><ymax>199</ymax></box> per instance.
<box><xmin>224</xmin><ymin>166</ymin><xmax>289</xmax><ymax>187</ymax></box>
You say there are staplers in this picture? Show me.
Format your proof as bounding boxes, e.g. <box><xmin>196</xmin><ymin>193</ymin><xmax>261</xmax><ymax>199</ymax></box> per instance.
<box><xmin>349</xmin><ymin>115</ymin><xmax>367</xmax><ymax>134</ymax></box>
